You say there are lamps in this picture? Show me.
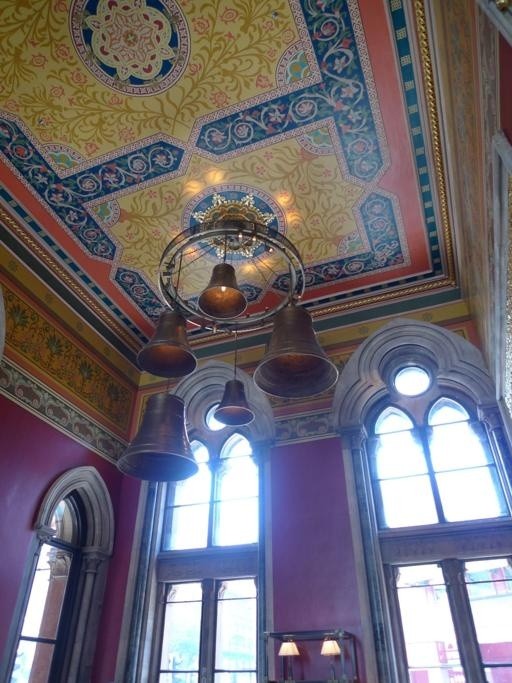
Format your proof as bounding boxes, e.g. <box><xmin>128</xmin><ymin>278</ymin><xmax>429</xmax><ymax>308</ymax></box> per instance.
<box><xmin>116</xmin><ymin>218</ymin><xmax>339</xmax><ymax>483</ymax></box>
<box><xmin>278</xmin><ymin>642</ymin><xmax>300</xmax><ymax>682</ymax></box>
<box><xmin>321</xmin><ymin>640</ymin><xmax>341</xmax><ymax>683</ymax></box>
